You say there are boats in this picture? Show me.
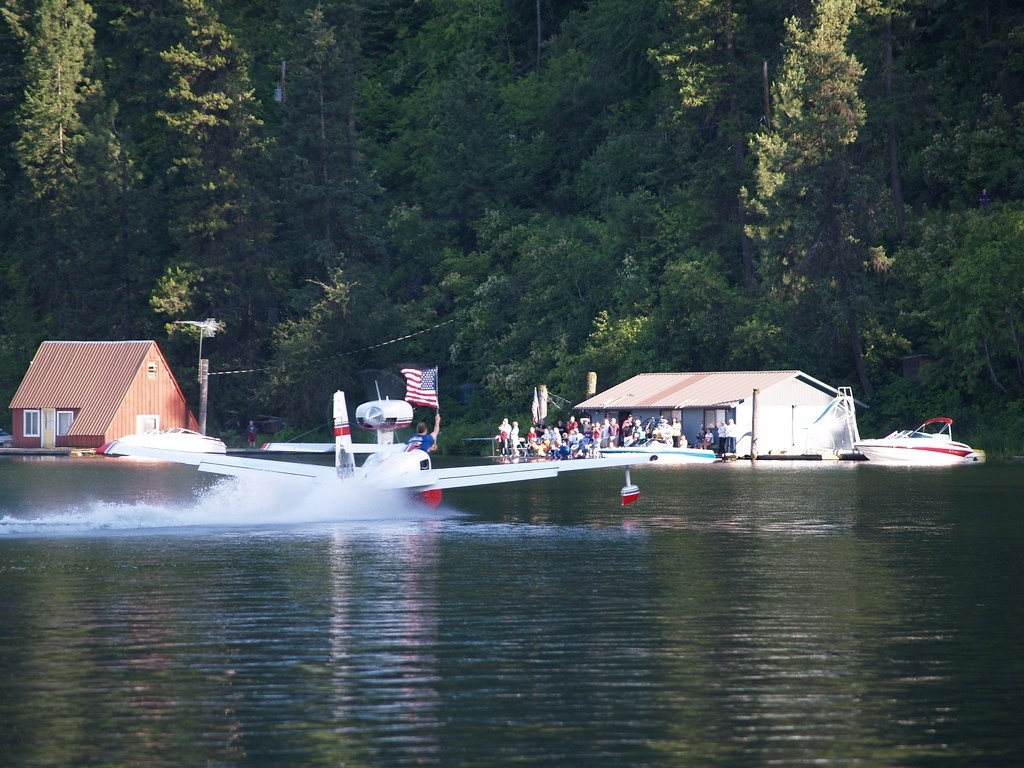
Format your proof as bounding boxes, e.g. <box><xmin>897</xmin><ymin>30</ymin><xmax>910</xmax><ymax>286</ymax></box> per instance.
<box><xmin>198</xmin><ymin>389</ymin><xmax>559</xmax><ymax>505</ymax></box>
<box><xmin>598</xmin><ymin>447</ymin><xmax>715</xmax><ymax>464</ymax></box>
<box><xmin>96</xmin><ymin>426</ymin><xmax>227</xmax><ymax>456</ymax></box>
<box><xmin>854</xmin><ymin>417</ymin><xmax>985</xmax><ymax>468</ymax></box>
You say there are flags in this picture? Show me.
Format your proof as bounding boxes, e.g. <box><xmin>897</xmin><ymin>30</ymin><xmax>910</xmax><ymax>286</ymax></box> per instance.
<box><xmin>531</xmin><ymin>388</ymin><xmax>539</xmax><ymax>425</ymax></box>
<box><xmin>393</xmin><ymin>363</ymin><xmax>440</xmax><ymax>410</ymax></box>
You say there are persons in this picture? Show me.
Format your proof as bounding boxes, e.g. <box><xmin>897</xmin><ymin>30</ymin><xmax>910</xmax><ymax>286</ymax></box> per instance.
<box><xmin>246</xmin><ymin>420</ymin><xmax>257</xmax><ymax>448</ymax></box>
<box><xmin>495</xmin><ymin>413</ymin><xmax>738</xmax><ymax>458</ymax></box>
<box><xmin>403</xmin><ymin>414</ymin><xmax>440</xmax><ymax>452</ymax></box>
